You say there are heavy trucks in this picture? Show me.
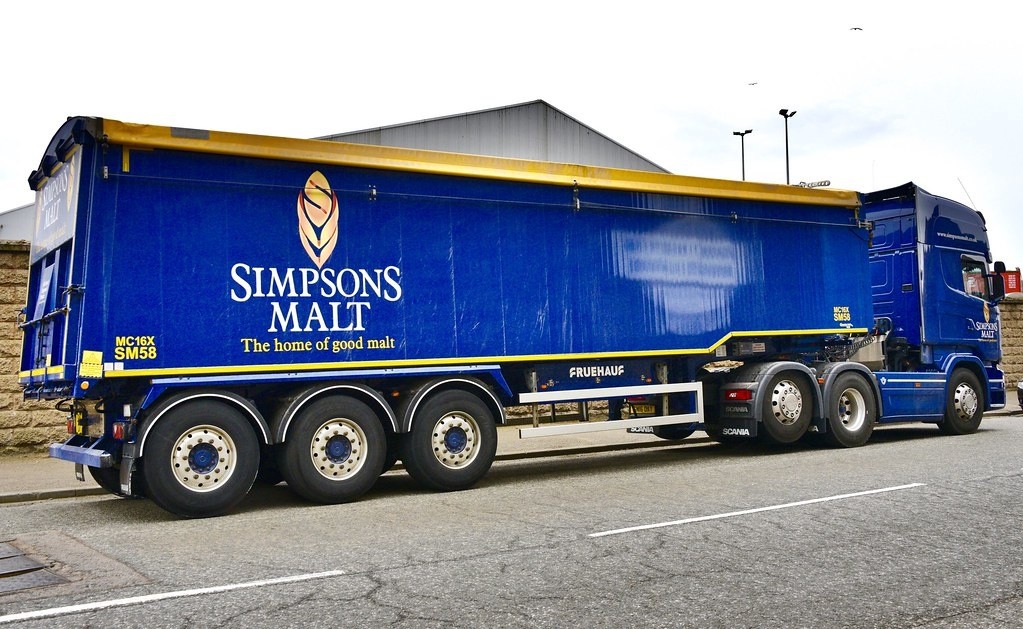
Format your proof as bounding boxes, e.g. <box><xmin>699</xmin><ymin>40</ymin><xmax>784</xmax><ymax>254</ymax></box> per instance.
<box><xmin>17</xmin><ymin>113</ymin><xmax>1003</xmax><ymax>519</ymax></box>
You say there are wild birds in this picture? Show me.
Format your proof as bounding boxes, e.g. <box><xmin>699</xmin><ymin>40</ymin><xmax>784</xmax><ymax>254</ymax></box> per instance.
<box><xmin>850</xmin><ymin>27</ymin><xmax>863</xmax><ymax>31</ymax></box>
<box><xmin>749</xmin><ymin>82</ymin><xmax>757</xmax><ymax>87</ymax></box>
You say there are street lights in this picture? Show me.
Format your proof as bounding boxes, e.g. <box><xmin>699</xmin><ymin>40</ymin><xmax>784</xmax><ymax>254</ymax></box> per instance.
<box><xmin>779</xmin><ymin>109</ymin><xmax>796</xmax><ymax>185</ymax></box>
<box><xmin>733</xmin><ymin>130</ymin><xmax>752</xmax><ymax>181</ymax></box>
